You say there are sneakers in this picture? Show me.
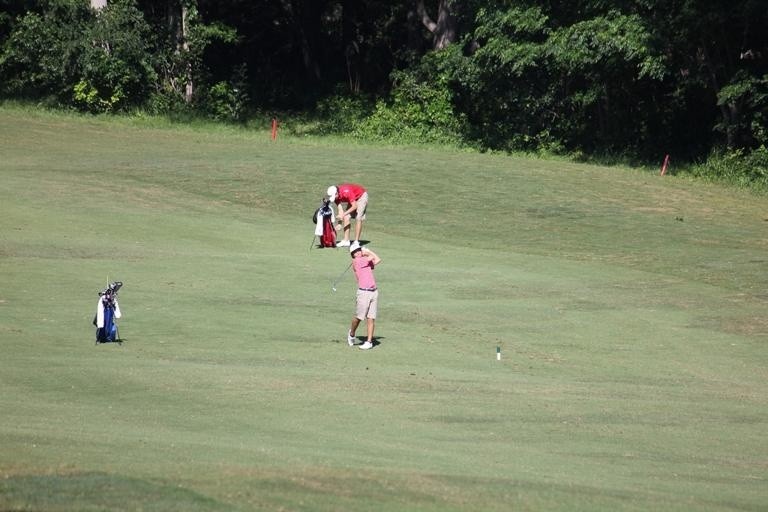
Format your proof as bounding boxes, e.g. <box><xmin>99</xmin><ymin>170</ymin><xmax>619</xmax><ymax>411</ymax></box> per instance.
<box><xmin>336</xmin><ymin>240</ymin><xmax>360</xmax><ymax>247</ymax></box>
<box><xmin>348</xmin><ymin>329</ymin><xmax>373</xmax><ymax>350</ymax></box>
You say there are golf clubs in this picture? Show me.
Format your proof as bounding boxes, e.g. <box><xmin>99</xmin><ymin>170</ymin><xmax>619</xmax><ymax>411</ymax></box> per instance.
<box><xmin>98</xmin><ymin>281</ymin><xmax>122</xmax><ymax>309</ymax></box>
<box><xmin>332</xmin><ymin>262</ymin><xmax>354</xmax><ymax>291</ymax></box>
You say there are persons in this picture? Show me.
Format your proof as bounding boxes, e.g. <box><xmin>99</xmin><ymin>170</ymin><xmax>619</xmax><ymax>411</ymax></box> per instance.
<box><xmin>347</xmin><ymin>242</ymin><xmax>381</xmax><ymax>349</ymax></box>
<box><xmin>312</xmin><ymin>198</ymin><xmax>339</xmax><ymax>249</ymax></box>
<box><xmin>95</xmin><ymin>288</ymin><xmax>122</xmax><ymax>343</ymax></box>
<box><xmin>326</xmin><ymin>182</ymin><xmax>369</xmax><ymax>252</ymax></box>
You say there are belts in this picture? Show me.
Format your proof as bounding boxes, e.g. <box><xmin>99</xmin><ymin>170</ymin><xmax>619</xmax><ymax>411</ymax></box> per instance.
<box><xmin>359</xmin><ymin>288</ymin><xmax>377</xmax><ymax>291</ymax></box>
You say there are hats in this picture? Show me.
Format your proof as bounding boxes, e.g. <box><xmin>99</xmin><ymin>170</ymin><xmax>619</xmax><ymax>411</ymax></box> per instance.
<box><xmin>327</xmin><ymin>186</ymin><xmax>337</xmax><ymax>202</ymax></box>
<box><xmin>350</xmin><ymin>243</ymin><xmax>361</xmax><ymax>252</ymax></box>
